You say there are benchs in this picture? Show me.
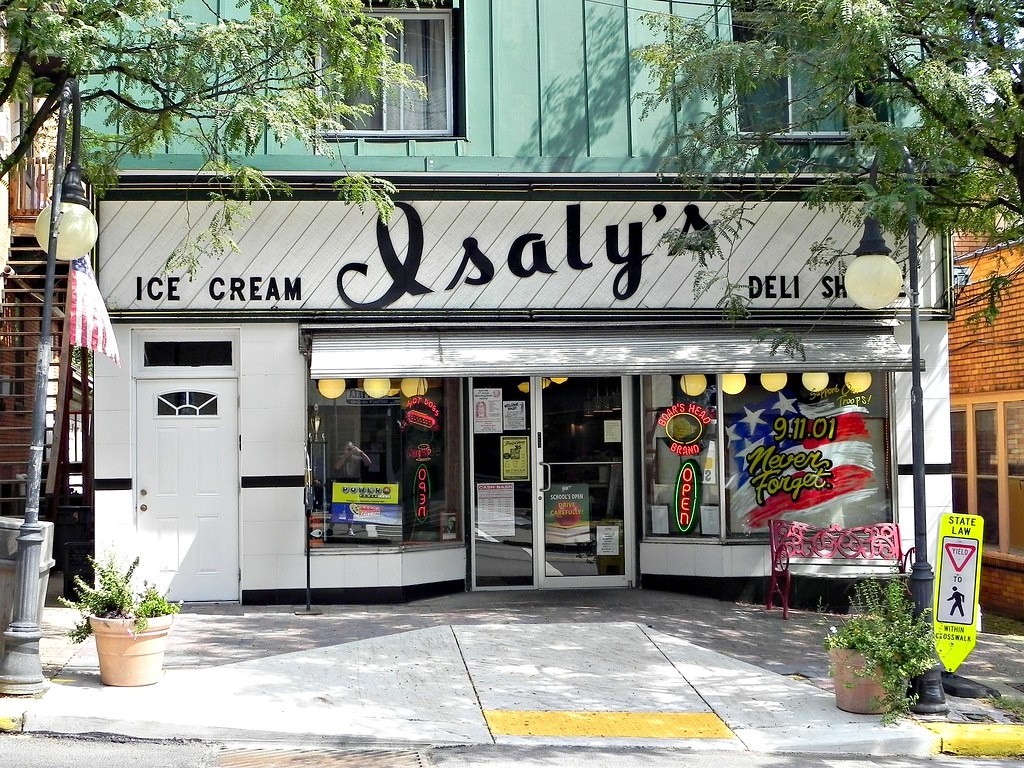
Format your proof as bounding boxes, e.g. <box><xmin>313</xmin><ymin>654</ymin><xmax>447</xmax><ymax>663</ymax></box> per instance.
<box><xmin>765</xmin><ymin>519</ymin><xmax>916</xmax><ymax>618</ymax></box>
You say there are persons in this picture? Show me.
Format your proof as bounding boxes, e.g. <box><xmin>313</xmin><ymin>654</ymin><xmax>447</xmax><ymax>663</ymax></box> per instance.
<box><xmin>325</xmin><ymin>441</ymin><xmax>371</xmax><ymax>536</ymax></box>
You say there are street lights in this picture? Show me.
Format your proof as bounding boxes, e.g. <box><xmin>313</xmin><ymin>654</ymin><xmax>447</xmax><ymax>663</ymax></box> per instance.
<box><xmin>844</xmin><ymin>145</ymin><xmax>953</xmax><ymax>716</ymax></box>
<box><xmin>0</xmin><ymin>76</ymin><xmax>100</xmax><ymax>696</ymax></box>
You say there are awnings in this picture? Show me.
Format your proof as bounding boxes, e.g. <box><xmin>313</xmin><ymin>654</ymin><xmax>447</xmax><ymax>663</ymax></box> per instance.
<box><xmin>309</xmin><ymin>331</ymin><xmax>925</xmax><ymax>379</ymax></box>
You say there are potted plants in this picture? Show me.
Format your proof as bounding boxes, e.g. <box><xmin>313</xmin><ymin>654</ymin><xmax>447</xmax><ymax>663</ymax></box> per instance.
<box><xmin>811</xmin><ymin>558</ymin><xmax>941</xmax><ymax>733</ymax></box>
<box><xmin>57</xmin><ymin>539</ymin><xmax>185</xmax><ymax>687</ymax></box>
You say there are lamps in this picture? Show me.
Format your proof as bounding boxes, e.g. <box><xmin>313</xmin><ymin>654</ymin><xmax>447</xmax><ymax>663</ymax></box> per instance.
<box><xmin>802</xmin><ymin>373</ymin><xmax>829</xmax><ymax>392</ymax></box>
<box><xmin>761</xmin><ymin>373</ymin><xmax>788</xmax><ymax>393</ymax></box>
<box><xmin>722</xmin><ymin>374</ymin><xmax>746</xmax><ymax>395</ymax></box>
<box><xmin>680</xmin><ymin>375</ymin><xmax>707</xmax><ymax>396</ymax></box>
<box><xmin>317</xmin><ymin>376</ymin><xmax>621</xmax><ymax>416</ymax></box>
<box><xmin>844</xmin><ymin>373</ymin><xmax>872</xmax><ymax>393</ymax></box>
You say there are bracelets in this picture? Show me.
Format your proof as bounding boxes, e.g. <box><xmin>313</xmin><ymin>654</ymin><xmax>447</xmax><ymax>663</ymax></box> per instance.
<box><xmin>360</xmin><ymin>451</ymin><xmax>363</xmax><ymax>457</ymax></box>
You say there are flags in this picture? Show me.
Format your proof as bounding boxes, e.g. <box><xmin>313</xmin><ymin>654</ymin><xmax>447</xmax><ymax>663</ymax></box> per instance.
<box><xmin>69</xmin><ymin>255</ymin><xmax>121</xmax><ymax>368</ymax></box>
